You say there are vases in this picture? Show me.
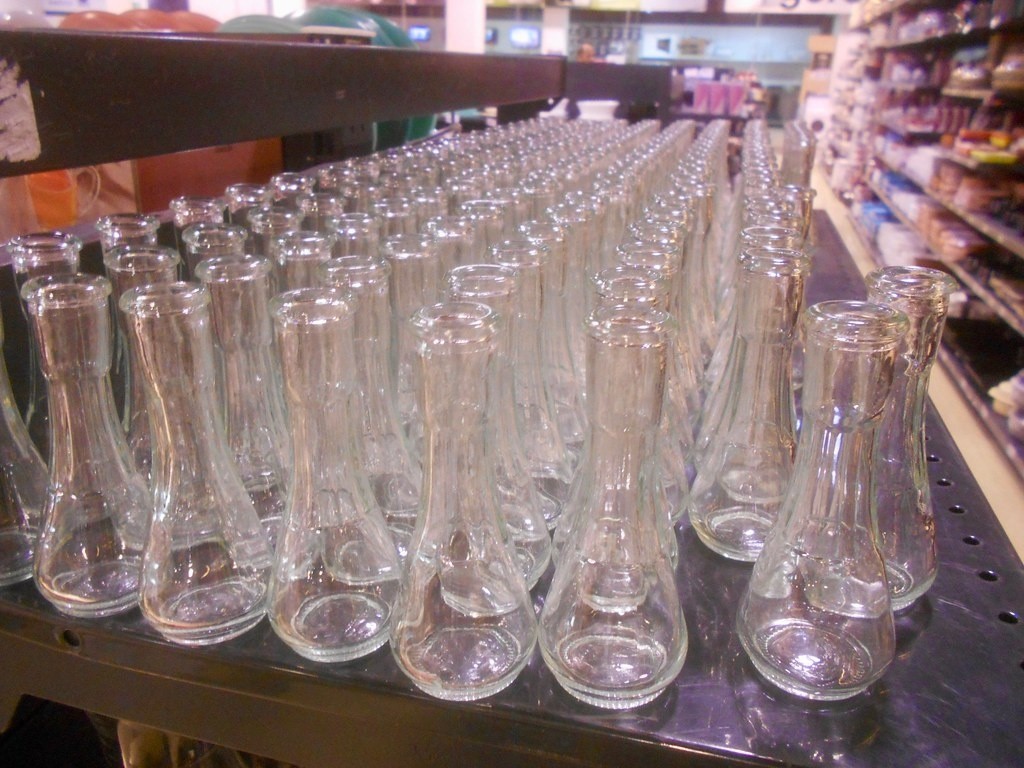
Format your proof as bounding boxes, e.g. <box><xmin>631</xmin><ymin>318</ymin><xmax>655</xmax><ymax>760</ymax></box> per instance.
<box><xmin>0</xmin><ymin>115</ymin><xmax>957</xmax><ymax>712</ymax></box>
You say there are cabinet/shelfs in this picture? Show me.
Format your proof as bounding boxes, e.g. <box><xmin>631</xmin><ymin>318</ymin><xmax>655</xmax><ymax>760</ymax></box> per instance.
<box><xmin>825</xmin><ymin>0</ymin><xmax>1024</xmax><ymax>488</ymax></box>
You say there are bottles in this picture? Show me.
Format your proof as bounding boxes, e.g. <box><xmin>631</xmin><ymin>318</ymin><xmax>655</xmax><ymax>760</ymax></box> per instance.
<box><xmin>0</xmin><ymin>116</ymin><xmax>956</xmax><ymax>709</ymax></box>
